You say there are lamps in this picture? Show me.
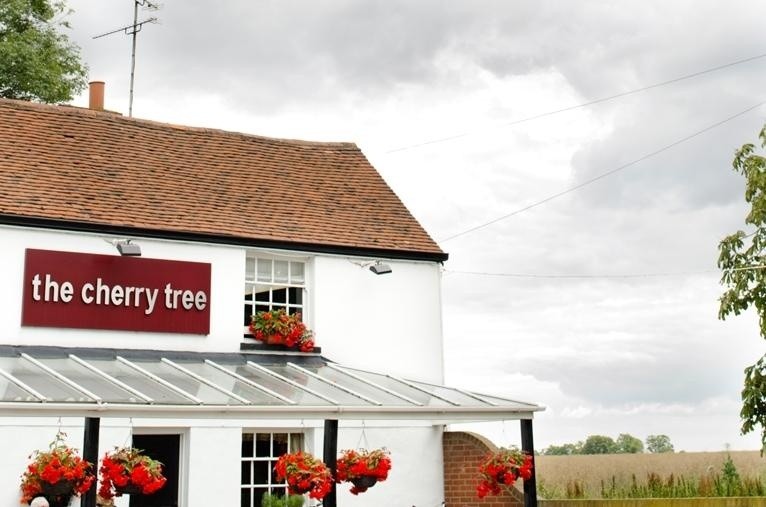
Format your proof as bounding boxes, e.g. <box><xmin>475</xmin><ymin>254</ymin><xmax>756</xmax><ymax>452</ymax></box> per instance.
<box><xmin>104</xmin><ymin>237</ymin><xmax>141</xmax><ymax>258</ymax></box>
<box><xmin>348</xmin><ymin>257</ymin><xmax>392</xmax><ymax>275</ymax></box>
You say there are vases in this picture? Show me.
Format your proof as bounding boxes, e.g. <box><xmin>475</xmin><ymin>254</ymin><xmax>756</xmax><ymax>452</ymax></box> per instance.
<box><xmin>350</xmin><ymin>473</ymin><xmax>377</xmax><ymax>488</ymax></box>
<box><xmin>112</xmin><ymin>479</ymin><xmax>143</xmax><ymax>495</ymax></box>
<box><xmin>41</xmin><ymin>479</ymin><xmax>76</xmax><ymax>506</ymax></box>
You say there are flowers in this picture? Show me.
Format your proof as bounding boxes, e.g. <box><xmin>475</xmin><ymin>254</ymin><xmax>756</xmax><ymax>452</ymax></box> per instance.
<box><xmin>273</xmin><ymin>449</ymin><xmax>336</xmax><ymax>500</ymax></box>
<box><xmin>249</xmin><ymin>308</ymin><xmax>315</xmax><ymax>351</ymax></box>
<box><xmin>474</xmin><ymin>442</ymin><xmax>535</xmax><ymax>499</ymax></box>
<box><xmin>16</xmin><ymin>431</ymin><xmax>97</xmax><ymax>504</ymax></box>
<box><xmin>334</xmin><ymin>446</ymin><xmax>393</xmax><ymax>494</ymax></box>
<box><xmin>99</xmin><ymin>445</ymin><xmax>168</xmax><ymax>495</ymax></box>
